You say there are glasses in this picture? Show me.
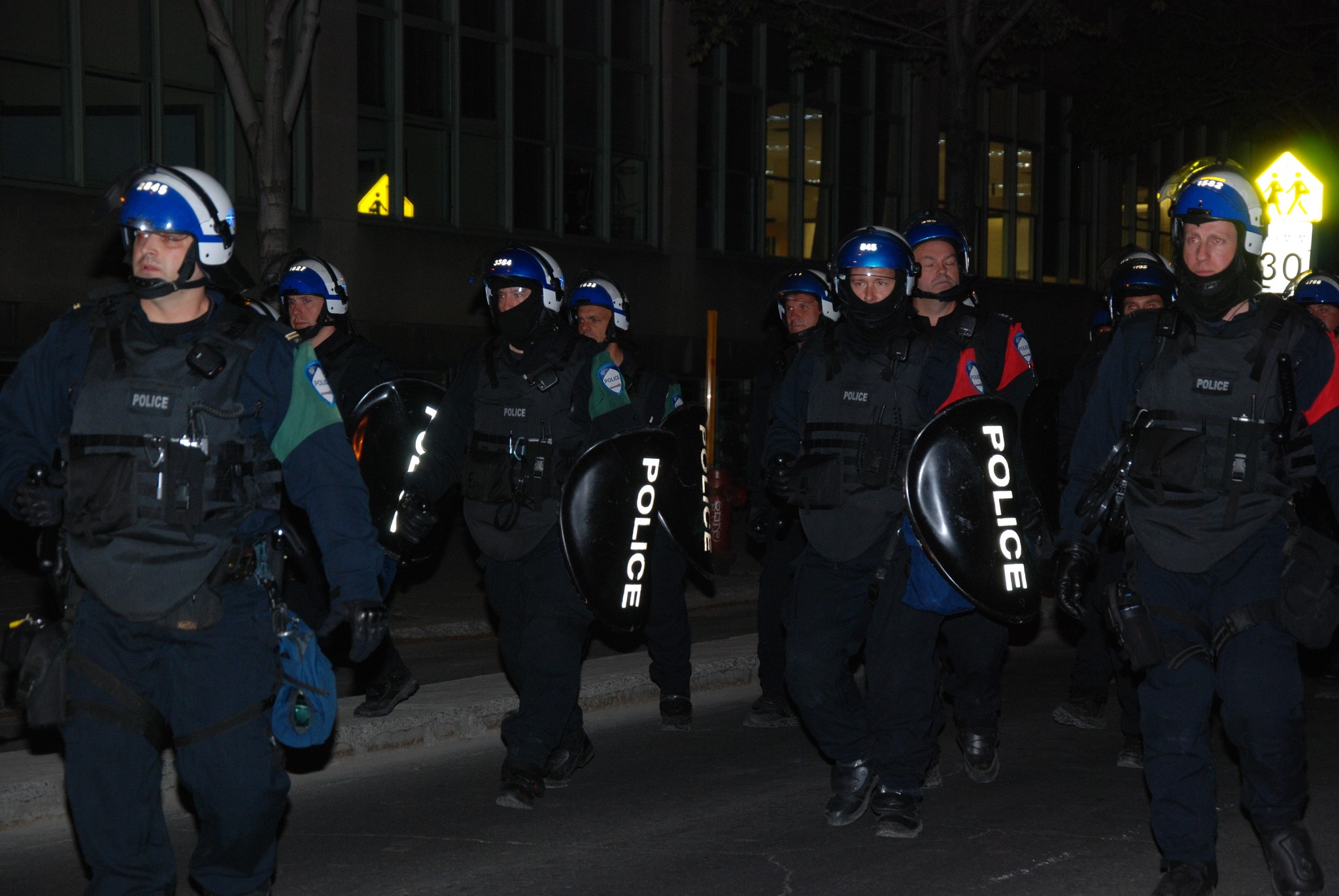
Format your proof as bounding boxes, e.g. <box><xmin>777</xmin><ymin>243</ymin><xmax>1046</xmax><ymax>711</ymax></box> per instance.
<box><xmin>134</xmin><ymin>229</ymin><xmax>191</xmax><ymax>249</ymax></box>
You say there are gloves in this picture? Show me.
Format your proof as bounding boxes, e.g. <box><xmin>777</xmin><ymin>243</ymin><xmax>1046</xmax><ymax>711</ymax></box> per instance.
<box><xmin>15</xmin><ymin>463</ymin><xmax>69</xmax><ymax>526</ymax></box>
<box><xmin>1052</xmin><ymin>542</ymin><xmax>1096</xmax><ymax>621</ymax></box>
<box><xmin>317</xmin><ymin>600</ymin><xmax>392</xmax><ymax>663</ymax></box>
<box><xmin>396</xmin><ymin>486</ymin><xmax>438</xmax><ymax>545</ymax></box>
<box><xmin>765</xmin><ymin>452</ymin><xmax>795</xmax><ymax>496</ymax></box>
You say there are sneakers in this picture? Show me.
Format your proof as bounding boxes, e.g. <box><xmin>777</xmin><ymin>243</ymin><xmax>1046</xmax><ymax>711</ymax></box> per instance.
<box><xmin>544</xmin><ymin>729</ymin><xmax>595</xmax><ymax>786</ymax></box>
<box><xmin>353</xmin><ymin>663</ymin><xmax>419</xmax><ymax>719</ymax></box>
<box><xmin>656</xmin><ymin>677</ymin><xmax>1144</xmax><ymax>837</ymax></box>
<box><xmin>1152</xmin><ymin>862</ymin><xmax>1219</xmax><ymax>896</ymax></box>
<box><xmin>496</xmin><ymin>780</ymin><xmax>545</xmax><ymax>811</ymax></box>
<box><xmin>1240</xmin><ymin>801</ymin><xmax>1325</xmax><ymax>896</ymax></box>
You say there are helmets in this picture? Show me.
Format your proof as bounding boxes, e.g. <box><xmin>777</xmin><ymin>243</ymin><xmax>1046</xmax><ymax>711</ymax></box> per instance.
<box><xmin>776</xmin><ymin>269</ymin><xmax>842</xmax><ymax>321</ymax></box>
<box><xmin>240</xmin><ymin>297</ymin><xmax>281</xmax><ymax>324</ymax></box>
<box><xmin>1108</xmin><ymin>248</ymin><xmax>1180</xmax><ymax>322</ymax></box>
<box><xmin>119</xmin><ymin>161</ymin><xmax>237</xmax><ymax>264</ymax></box>
<box><xmin>278</xmin><ymin>249</ymin><xmax>348</xmax><ymax>314</ymax></box>
<box><xmin>570</xmin><ymin>277</ymin><xmax>632</xmax><ymax>331</ymax></box>
<box><xmin>1168</xmin><ymin>162</ymin><xmax>1268</xmax><ymax>256</ymax></box>
<box><xmin>900</xmin><ymin>210</ymin><xmax>976</xmax><ymax>301</ymax></box>
<box><xmin>1294</xmin><ymin>269</ymin><xmax>1339</xmax><ymax>304</ymax></box>
<box><xmin>833</xmin><ymin>225</ymin><xmax>922</xmax><ymax>329</ymax></box>
<box><xmin>482</xmin><ymin>245</ymin><xmax>565</xmax><ymax>315</ymax></box>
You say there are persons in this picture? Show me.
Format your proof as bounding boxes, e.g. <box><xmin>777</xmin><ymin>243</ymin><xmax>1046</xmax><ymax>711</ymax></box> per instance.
<box><xmin>279</xmin><ymin>242</ymin><xmax>699</xmax><ymax>811</ymax></box>
<box><xmin>744</xmin><ymin>162</ymin><xmax>1339</xmax><ymax>895</ymax></box>
<box><xmin>0</xmin><ymin>165</ymin><xmax>399</xmax><ymax>896</ymax></box>
